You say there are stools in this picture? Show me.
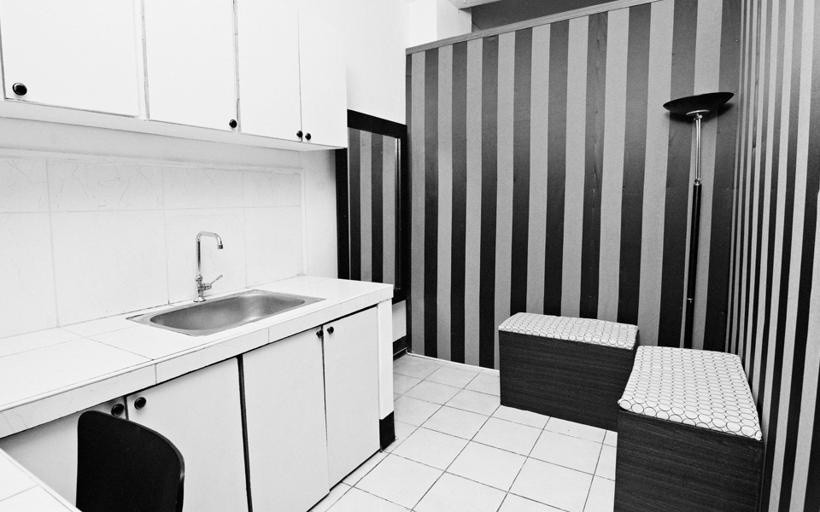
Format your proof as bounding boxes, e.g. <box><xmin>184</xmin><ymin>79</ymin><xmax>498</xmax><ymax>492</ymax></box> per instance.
<box><xmin>497</xmin><ymin>312</ymin><xmax>639</xmax><ymax>433</ymax></box>
<box><xmin>614</xmin><ymin>345</ymin><xmax>767</xmax><ymax>511</ymax></box>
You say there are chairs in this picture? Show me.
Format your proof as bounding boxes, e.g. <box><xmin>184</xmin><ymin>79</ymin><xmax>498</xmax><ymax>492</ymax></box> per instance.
<box><xmin>76</xmin><ymin>411</ymin><xmax>183</xmax><ymax>512</ymax></box>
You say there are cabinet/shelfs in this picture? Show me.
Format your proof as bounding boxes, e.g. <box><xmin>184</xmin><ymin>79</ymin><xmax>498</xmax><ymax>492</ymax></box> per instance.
<box><xmin>235</xmin><ymin>1</ymin><xmax>349</xmax><ymax>152</ymax></box>
<box><xmin>238</xmin><ymin>307</ymin><xmax>379</xmax><ymax>511</ymax></box>
<box><xmin>0</xmin><ymin>357</ymin><xmax>248</xmax><ymax>512</ymax></box>
<box><xmin>1</xmin><ymin>0</ymin><xmax>145</xmax><ymax>131</ymax></box>
<box><xmin>141</xmin><ymin>0</ymin><xmax>237</xmax><ymax>142</ymax></box>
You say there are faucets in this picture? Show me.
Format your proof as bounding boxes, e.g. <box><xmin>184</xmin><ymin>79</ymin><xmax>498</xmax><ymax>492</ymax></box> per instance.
<box><xmin>194</xmin><ymin>230</ymin><xmax>224</xmax><ymax>302</ymax></box>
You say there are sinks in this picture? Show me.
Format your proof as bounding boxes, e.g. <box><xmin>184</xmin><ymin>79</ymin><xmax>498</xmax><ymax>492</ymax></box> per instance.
<box><xmin>125</xmin><ymin>289</ymin><xmax>326</xmax><ymax>337</ymax></box>
